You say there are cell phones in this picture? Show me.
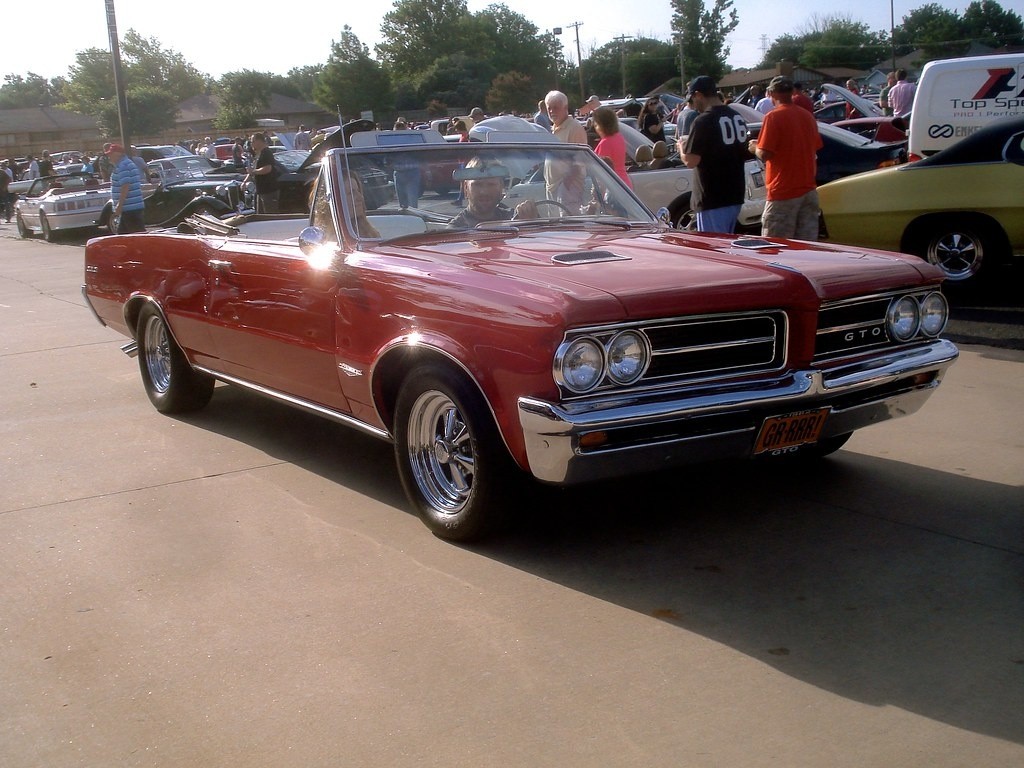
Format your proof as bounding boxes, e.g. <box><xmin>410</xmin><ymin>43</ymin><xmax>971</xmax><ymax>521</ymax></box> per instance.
<box><xmin>670</xmin><ymin>138</ymin><xmax>678</xmax><ymax>144</ymax></box>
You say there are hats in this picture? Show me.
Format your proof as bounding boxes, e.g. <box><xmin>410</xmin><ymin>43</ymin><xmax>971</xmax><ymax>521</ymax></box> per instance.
<box><xmin>104</xmin><ymin>144</ymin><xmax>123</xmax><ymax>155</ymax></box>
<box><xmin>468</xmin><ymin>107</ymin><xmax>483</xmax><ymax>117</ymax></box>
<box><xmin>395</xmin><ymin>117</ymin><xmax>406</xmax><ymax>124</ymax></box>
<box><xmin>681</xmin><ymin>76</ymin><xmax>715</xmax><ymax>104</ymax></box>
<box><xmin>585</xmin><ymin>95</ymin><xmax>599</xmax><ymax>103</ymax></box>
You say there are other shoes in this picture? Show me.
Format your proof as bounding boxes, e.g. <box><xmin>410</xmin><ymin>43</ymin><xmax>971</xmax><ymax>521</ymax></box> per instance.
<box><xmin>5</xmin><ymin>217</ymin><xmax>11</xmax><ymax>223</ymax></box>
<box><xmin>450</xmin><ymin>200</ymin><xmax>462</xmax><ymax>206</ymax></box>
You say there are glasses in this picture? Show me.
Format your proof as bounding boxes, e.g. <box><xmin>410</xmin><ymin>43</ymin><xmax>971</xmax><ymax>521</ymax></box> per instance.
<box><xmin>648</xmin><ymin>102</ymin><xmax>657</xmax><ymax>105</ymax></box>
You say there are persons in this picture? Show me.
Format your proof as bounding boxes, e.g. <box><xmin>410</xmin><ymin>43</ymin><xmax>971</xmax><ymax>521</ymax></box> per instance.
<box><xmin>95</xmin><ymin>128</ymin><xmax>329</xmax><ymax>180</ymax></box>
<box><xmin>302</xmin><ymin>169</ymin><xmax>379</xmax><ymax>244</ymax></box>
<box><xmin>106</xmin><ymin>144</ymin><xmax>146</xmax><ymax>234</ymax></box>
<box><xmin>246</xmin><ymin>132</ymin><xmax>283</xmax><ymax>214</ymax></box>
<box><xmin>445</xmin><ymin>155</ymin><xmax>539</xmax><ymax>229</ymax></box>
<box><xmin>719</xmin><ymin>80</ymin><xmax>869</xmax><ymax>113</ymax></box>
<box><xmin>294</xmin><ymin>125</ymin><xmax>311</xmax><ymax>149</ymax></box>
<box><xmin>879</xmin><ymin>68</ymin><xmax>916</xmax><ymax>116</ymax></box>
<box><xmin>747</xmin><ymin>76</ymin><xmax>820</xmax><ymax>240</ymax></box>
<box><xmin>845</xmin><ymin>80</ymin><xmax>860</xmax><ymax>119</ymax></box>
<box><xmin>534</xmin><ymin>76</ymin><xmax>749</xmax><ymax>235</ymax></box>
<box><xmin>391</xmin><ymin>106</ymin><xmax>533</xmax><ymax>207</ymax></box>
<box><xmin>0</xmin><ymin>150</ymin><xmax>105</xmax><ymax>223</ymax></box>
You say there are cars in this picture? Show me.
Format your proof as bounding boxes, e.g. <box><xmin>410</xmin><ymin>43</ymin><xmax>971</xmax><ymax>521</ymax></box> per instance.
<box><xmin>830</xmin><ymin>117</ymin><xmax>909</xmax><ymax>142</ymax></box>
<box><xmin>3</xmin><ymin>144</ymin><xmax>243</xmax><ymax>194</ymax></box>
<box><xmin>418</xmin><ymin>134</ymin><xmax>468</xmax><ymax>197</ymax></box>
<box><xmin>814</xmin><ymin>98</ymin><xmax>881</xmax><ymax>125</ymax></box>
<box><xmin>147</xmin><ymin>155</ymin><xmax>219</xmax><ymax>183</ymax></box>
<box><xmin>141</xmin><ymin>167</ymin><xmax>256</xmax><ymax>228</ymax></box>
<box><xmin>81</xmin><ymin>129</ymin><xmax>960</xmax><ymax>546</ymax></box>
<box><xmin>203</xmin><ymin>118</ymin><xmax>395</xmax><ymax>213</ymax></box>
<box><xmin>668</xmin><ymin>121</ymin><xmax>909</xmax><ymax>187</ymax></box>
<box><xmin>815</xmin><ymin>113</ymin><xmax>1024</xmax><ymax>305</ymax></box>
<box><xmin>14</xmin><ymin>172</ymin><xmax>111</xmax><ymax>243</ymax></box>
<box><xmin>468</xmin><ymin>115</ymin><xmax>768</xmax><ymax>234</ymax></box>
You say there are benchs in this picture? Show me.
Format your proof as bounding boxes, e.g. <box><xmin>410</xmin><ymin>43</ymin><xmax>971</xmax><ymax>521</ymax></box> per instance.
<box><xmin>224</xmin><ymin>213</ymin><xmax>428</xmax><ymax>244</ymax></box>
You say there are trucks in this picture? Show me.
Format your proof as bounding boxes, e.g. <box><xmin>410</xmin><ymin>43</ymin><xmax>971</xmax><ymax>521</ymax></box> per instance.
<box><xmin>908</xmin><ymin>53</ymin><xmax>1024</xmax><ymax>162</ymax></box>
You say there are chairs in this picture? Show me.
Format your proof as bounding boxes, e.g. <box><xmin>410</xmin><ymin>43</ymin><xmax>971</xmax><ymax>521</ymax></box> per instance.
<box><xmin>43</xmin><ymin>182</ymin><xmax>62</xmax><ymax>194</ymax></box>
<box><xmin>85</xmin><ymin>179</ymin><xmax>99</xmax><ymax>189</ymax></box>
<box><xmin>650</xmin><ymin>141</ymin><xmax>668</xmax><ymax>169</ymax></box>
<box><xmin>635</xmin><ymin>145</ymin><xmax>653</xmax><ymax>171</ymax></box>
